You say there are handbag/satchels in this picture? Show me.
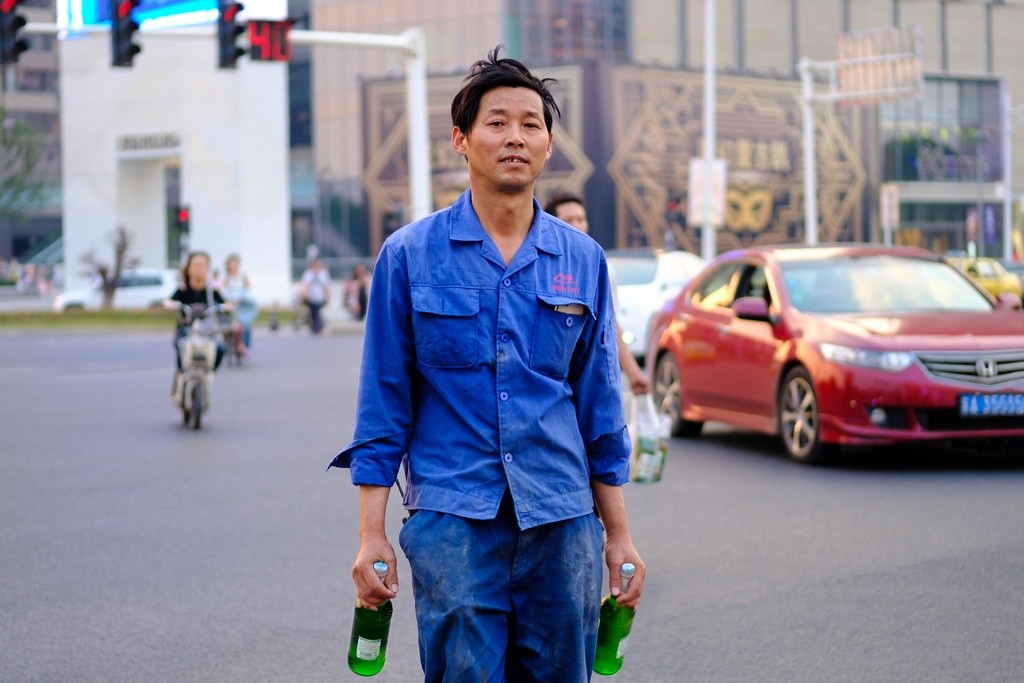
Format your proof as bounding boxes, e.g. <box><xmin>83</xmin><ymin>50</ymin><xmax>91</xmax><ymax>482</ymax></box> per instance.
<box><xmin>627</xmin><ymin>392</ymin><xmax>672</xmax><ymax>482</ymax></box>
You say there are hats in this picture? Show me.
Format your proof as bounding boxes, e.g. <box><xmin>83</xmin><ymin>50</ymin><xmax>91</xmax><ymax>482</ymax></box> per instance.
<box><xmin>305</xmin><ymin>243</ymin><xmax>320</xmax><ymax>261</ymax></box>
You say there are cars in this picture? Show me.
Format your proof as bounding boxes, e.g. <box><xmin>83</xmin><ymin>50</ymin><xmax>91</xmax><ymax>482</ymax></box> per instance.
<box><xmin>641</xmin><ymin>241</ymin><xmax>1024</xmax><ymax>467</ymax></box>
<box><xmin>948</xmin><ymin>252</ymin><xmax>1023</xmax><ymax>309</ymax></box>
<box><xmin>50</xmin><ymin>267</ymin><xmax>186</xmax><ymax>313</ymax></box>
<box><xmin>606</xmin><ymin>244</ymin><xmax>710</xmax><ymax>371</ymax></box>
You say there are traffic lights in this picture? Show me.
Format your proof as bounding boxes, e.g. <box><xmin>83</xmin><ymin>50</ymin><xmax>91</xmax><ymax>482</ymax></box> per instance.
<box><xmin>173</xmin><ymin>206</ymin><xmax>190</xmax><ymax>236</ymax></box>
<box><xmin>109</xmin><ymin>0</ymin><xmax>142</xmax><ymax>68</ymax></box>
<box><xmin>216</xmin><ymin>0</ymin><xmax>245</xmax><ymax>70</ymax></box>
<box><xmin>1</xmin><ymin>0</ymin><xmax>32</xmax><ymax>64</ymax></box>
<box><xmin>245</xmin><ymin>16</ymin><xmax>296</xmax><ymax>61</ymax></box>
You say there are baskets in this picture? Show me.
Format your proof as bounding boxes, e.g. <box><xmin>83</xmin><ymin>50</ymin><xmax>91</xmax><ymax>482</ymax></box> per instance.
<box><xmin>176</xmin><ymin>334</ymin><xmax>216</xmax><ymax>369</ymax></box>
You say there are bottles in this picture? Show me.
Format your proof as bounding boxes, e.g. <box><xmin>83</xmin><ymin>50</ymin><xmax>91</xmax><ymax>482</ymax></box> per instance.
<box><xmin>593</xmin><ymin>563</ymin><xmax>636</xmax><ymax>674</ymax></box>
<box><xmin>348</xmin><ymin>562</ymin><xmax>392</xmax><ymax>677</ymax></box>
<box><xmin>631</xmin><ymin>429</ymin><xmax>667</xmax><ymax>483</ymax></box>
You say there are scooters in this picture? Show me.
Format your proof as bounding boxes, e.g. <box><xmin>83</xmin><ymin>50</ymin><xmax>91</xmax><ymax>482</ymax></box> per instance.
<box><xmin>164</xmin><ymin>299</ymin><xmax>232</xmax><ymax>430</ymax></box>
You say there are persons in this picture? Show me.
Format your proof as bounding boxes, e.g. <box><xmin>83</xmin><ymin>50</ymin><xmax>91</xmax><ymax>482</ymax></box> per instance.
<box><xmin>327</xmin><ymin>43</ymin><xmax>652</xmax><ymax>682</ymax></box>
<box><xmin>342</xmin><ymin>264</ymin><xmax>370</xmax><ymax>320</ymax></box>
<box><xmin>293</xmin><ymin>258</ymin><xmax>331</xmax><ymax>335</ymax></box>
<box><xmin>167</xmin><ymin>250</ymin><xmax>256</xmax><ymax>394</ymax></box>
